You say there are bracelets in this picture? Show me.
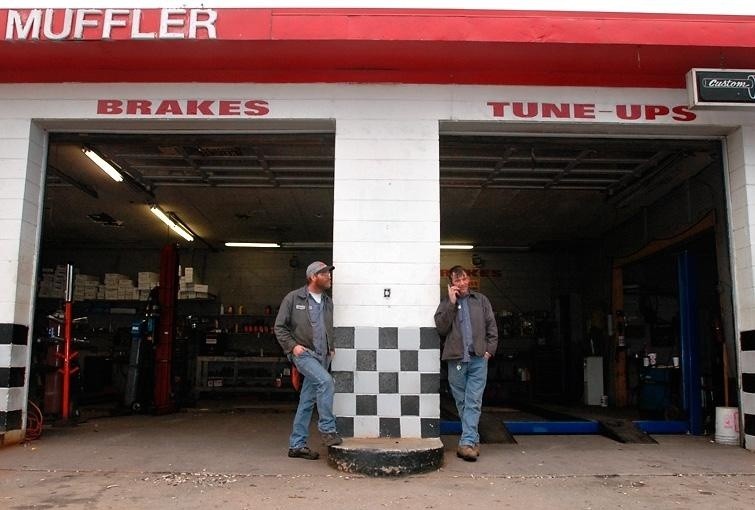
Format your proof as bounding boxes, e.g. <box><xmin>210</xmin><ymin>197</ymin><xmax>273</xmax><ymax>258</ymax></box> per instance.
<box><xmin>485</xmin><ymin>352</ymin><xmax>492</xmax><ymax>357</ymax></box>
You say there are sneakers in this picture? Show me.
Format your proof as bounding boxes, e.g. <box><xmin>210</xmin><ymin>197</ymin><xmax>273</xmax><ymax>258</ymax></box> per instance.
<box><xmin>320</xmin><ymin>431</ymin><xmax>343</xmax><ymax>447</ymax></box>
<box><xmin>457</xmin><ymin>442</ymin><xmax>480</xmax><ymax>462</ymax></box>
<box><xmin>287</xmin><ymin>447</ymin><xmax>319</xmax><ymax>460</ymax></box>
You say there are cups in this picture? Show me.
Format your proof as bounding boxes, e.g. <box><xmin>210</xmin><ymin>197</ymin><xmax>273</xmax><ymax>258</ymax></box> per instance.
<box><xmin>648</xmin><ymin>353</ymin><xmax>657</xmax><ymax>365</ymax></box>
<box><xmin>673</xmin><ymin>357</ymin><xmax>679</xmax><ymax>367</ymax></box>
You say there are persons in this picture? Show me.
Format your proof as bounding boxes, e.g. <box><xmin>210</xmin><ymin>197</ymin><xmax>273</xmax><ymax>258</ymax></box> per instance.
<box><xmin>273</xmin><ymin>260</ymin><xmax>342</xmax><ymax>458</ymax></box>
<box><xmin>433</xmin><ymin>265</ymin><xmax>500</xmax><ymax>461</ymax></box>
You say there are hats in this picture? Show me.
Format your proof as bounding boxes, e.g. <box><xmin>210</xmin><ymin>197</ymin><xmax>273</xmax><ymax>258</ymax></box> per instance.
<box><xmin>305</xmin><ymin>261</ymin><xmax>335</xmax><ymax>279</ymax></box>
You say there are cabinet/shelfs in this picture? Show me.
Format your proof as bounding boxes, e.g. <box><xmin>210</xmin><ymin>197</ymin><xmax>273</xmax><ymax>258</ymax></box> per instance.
<box><xmin>191</xmin><ymin>357</ymin><xmax>297</xmax><ymax>401</ymax></box>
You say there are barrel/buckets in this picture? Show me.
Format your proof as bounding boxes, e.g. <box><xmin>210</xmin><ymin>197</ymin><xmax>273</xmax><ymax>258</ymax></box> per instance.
<box><xmin>714</xmin><ymin>407</ymin><xmax>740</xmax><ymax>445</ymax></box>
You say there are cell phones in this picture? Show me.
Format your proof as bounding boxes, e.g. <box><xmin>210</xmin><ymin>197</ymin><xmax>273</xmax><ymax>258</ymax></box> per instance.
<box><xmin>448</xmin><ymin>281</ymin><xmax>454</xmax><ymax>287</ymax></box>
